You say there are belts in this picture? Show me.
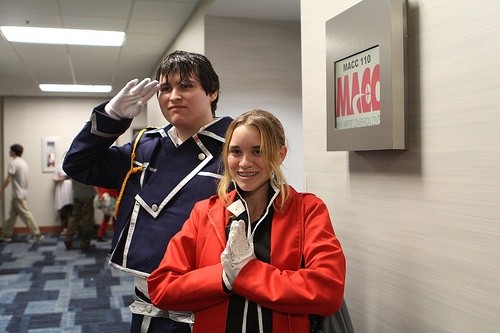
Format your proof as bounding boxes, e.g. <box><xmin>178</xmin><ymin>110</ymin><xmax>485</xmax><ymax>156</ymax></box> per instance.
<box><xmin>130</xmin><ymin>291</ymin><xmax>195</xmax><ymax>333</ymax></box>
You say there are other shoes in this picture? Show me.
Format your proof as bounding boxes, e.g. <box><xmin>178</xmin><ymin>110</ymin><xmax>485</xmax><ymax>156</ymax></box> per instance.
<box><xmin>0</xmin><ymin>235</ymin><xmax>13</xmax><ymax>241</ymax></box>
<box><xmin>81</xmin><ymin>239</ymin><xmax>96</xmax><ymax>251</ymax></box>
<box><xmin>97</xmin><ymin>236</ymin><xmax>107</xmax><ymax>241</ymax></box>
<box><xmin>28</xmin><ymin>232</ymin><xmax>45</xmax><ymax>243</ymax></box>
<box><xmin>60</xmin><ymin>228</ymin><xmax>69</xmax><ymax>235</ymax></box>
<box><xmin>64</xmin><ymin>239</ymin><xmax>72</xmax><ymax>250</ymax></box>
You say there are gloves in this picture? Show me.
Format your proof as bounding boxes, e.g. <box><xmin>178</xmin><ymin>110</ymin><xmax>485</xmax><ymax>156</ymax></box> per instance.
<box><xmin>232</xmin><ymin>219</ymin><xmax>257</xmax><ymax>276</ymax></box>
<box><xmin>220</xmin><ymin>220</ymin><xmax>238</xmax><ymax>283</ymax></box>
<box><xmin>104</xmin><ymin>77</ymin><xmax>161</xmax><ymax>119</ymax></box>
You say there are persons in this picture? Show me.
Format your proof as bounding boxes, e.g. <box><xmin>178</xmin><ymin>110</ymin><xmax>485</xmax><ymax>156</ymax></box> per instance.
<box><xmin>1</xmin><ymin>143</ymin><xmax>44</xmax><ymax>245</ymax></box>
<box><xmin>53</xmin><ymin>161</ymin><xmax>116</xmax><ymax>252</ymax></box>
<box><xmin>147</xmin><ymin>110</ymin><xmax>346</xmax><ymax>333</ymax></box>
<box><xmin>62</xmin><ymin>48</ymin><xmax>234</xmax><ymax>333</ymax></box>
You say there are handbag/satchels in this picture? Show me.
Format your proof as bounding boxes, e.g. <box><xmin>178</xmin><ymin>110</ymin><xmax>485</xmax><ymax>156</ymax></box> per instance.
<box><xmin>310</xmin><ymin>295</ymin><xmax>356</xmax><ymax>332</ymax></box>
